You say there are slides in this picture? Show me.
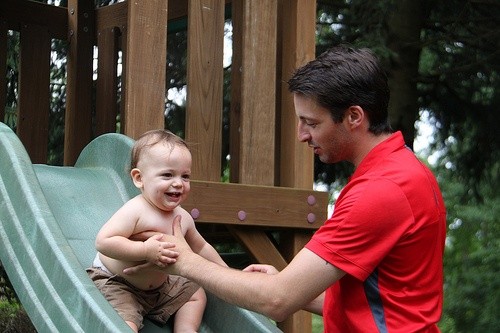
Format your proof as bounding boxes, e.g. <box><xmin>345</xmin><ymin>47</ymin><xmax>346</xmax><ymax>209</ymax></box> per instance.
<box><xmin>1</xmin><ymin>122</ymin><xmax>282</xmax><ymax>333</ymax></box>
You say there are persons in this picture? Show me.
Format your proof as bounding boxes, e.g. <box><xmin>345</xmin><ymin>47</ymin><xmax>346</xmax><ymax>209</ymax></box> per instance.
<box><xmin>84</xmin><ymin>128</ymin><xmax>233</xmax><ymax>333</ymax></box>
<box><xmin>121</xmin><ymin>47</ymin><xmax>447</xmax><ymax>333</ymax></box>
